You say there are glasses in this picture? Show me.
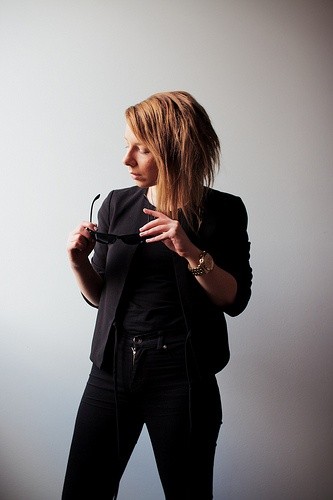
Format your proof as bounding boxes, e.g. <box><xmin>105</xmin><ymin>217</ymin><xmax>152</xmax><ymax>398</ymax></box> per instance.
<box><xmin>90</xmin><ymin>193</ymin><xmax>149</xmax><ymax>245</ymax></box>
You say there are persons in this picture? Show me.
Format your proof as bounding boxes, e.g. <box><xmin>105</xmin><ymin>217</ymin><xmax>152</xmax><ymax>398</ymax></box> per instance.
<box><xmin>61</xmin><ymin>90</ymin><xmax>253</xmax><ymax>500</ymax></box>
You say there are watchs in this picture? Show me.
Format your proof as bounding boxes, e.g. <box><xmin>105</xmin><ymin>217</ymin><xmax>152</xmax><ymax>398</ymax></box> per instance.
<box><xmin>188</xmin><ymin>252</ymin><xmax>214</xmax><ymax>275</ymax></box>
